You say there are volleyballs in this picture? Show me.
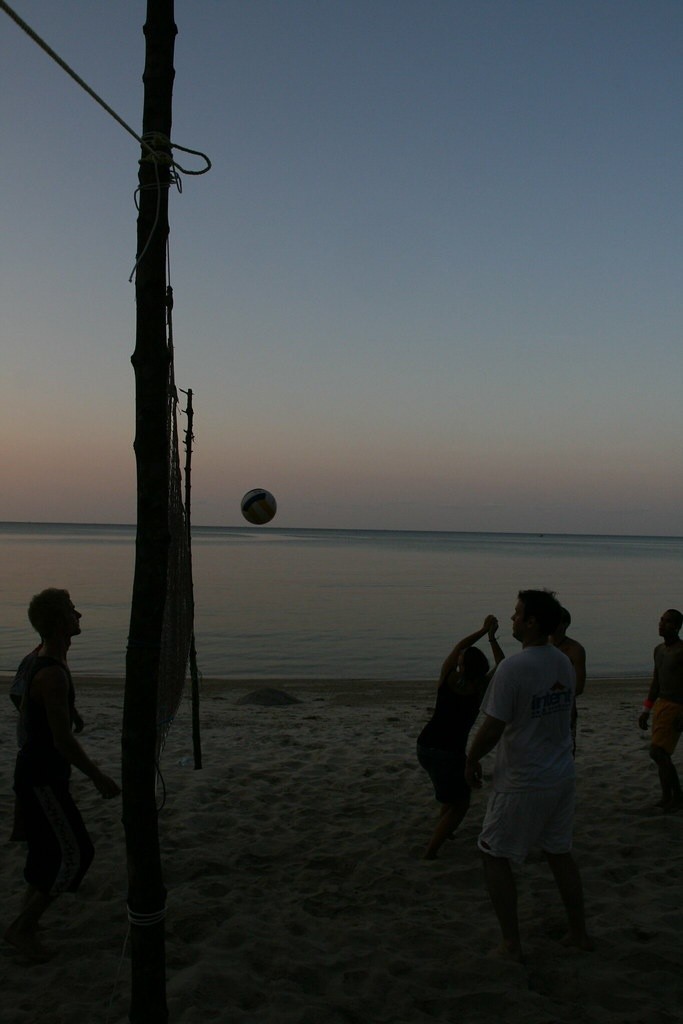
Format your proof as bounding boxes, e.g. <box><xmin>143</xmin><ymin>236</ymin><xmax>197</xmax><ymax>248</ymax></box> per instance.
<box><xmin>241</xmin><ymin>488</ymin><xmax>277</xmax><ymax>525</ymax></box>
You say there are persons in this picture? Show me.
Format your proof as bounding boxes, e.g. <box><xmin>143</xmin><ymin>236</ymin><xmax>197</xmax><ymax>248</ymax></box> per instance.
<box><xmin>416</xmin><ymin>590</ymin><xmax>596</xmax><ymax>961</ymax></box>
<box><xmin>639</xmin><ymin>609</ymin><xmax>683</xmax><ymax>810</ymax></box>
<box><xmin>2</xmin><ymin>589</ymin><xmax>122</xmax><ymax>962</ymax></box>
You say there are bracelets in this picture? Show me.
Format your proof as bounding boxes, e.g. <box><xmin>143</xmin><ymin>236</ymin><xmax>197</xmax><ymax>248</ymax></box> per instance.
<box><xmin>642</xmin><ymin>698</ymin><xmax>654</xmax><ymax>714</ymax></box>
